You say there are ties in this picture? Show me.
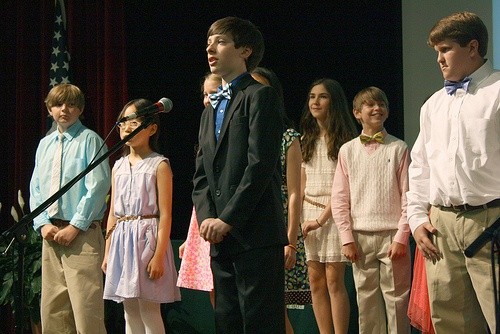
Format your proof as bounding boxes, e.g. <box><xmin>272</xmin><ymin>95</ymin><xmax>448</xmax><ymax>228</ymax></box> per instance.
<box><xmin>47</xmin><ymin>134</ymin><xmax>66</xmax><ymax>218</ymax></box>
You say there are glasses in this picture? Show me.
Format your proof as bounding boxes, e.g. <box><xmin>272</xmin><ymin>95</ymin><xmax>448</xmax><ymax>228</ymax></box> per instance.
<box><xmin>116</xmin><ymin>121</ymin><xmax>140</xmax><ymax>129</ymax></box>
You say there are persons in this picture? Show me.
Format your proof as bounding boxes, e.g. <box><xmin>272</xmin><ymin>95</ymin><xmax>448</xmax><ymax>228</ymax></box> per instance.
<box><xmin>102</xmin><ymin>99</ymin><xmax>181</xmax><ymax>334</ymax></box>
<box><xmin>29</xmin><ymin>85</ymin><xmax>110</xmax><ymax>334</ymax></box>
<box><xmin>299</xmin><ymin>78</ymin><xmax>353</xmax><ymax>334</ymax></box>
<box><xmin>330</xmin><ymin>86</ymin><xmax>412</xmax><ymax>334</ymax></box>
<box><xmin>175</xmin><ymin>66</ymin><xmax>311</xmax><ymax>334</ymax></box>
<box><xmin>191</xmin><ymin>18</ymin><xmax>288</xmax><ymax>334</ymax></box>
<box><xmin>404</xmin><ymin>10</ymin><xmax>500</xmax><ymax>334</ymax></box>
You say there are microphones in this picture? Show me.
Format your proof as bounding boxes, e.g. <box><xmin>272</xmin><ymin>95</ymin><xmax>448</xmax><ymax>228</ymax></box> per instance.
<box><xmin>120</xmin><ymin>97</ymin><xmax>174</xmax><ymax>122</ymax></box>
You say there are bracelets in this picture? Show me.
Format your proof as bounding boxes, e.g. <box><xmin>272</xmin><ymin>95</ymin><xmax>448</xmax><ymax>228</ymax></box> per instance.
<box><xmin>288</xmin><ymin>243</ymin><xmax>297</xmax><ymax>251</ymax></box>
<box><xmin>316</xmin><ymin>219</ymin><xmax>322</xmax><ymax>227</ymax></box>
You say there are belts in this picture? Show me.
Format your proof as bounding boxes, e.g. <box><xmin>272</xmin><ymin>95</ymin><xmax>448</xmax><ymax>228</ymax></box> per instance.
<box><xmin>105</xmin><ymin>215</ymin><xmax>158</xmax><ymax>240</ymax></box>
<box><xmin>49</xmin><ymin>220</ymin><xmax>97</xmax><ymax>229</ymax></box>
<box><xmin>442</xmin><ymin>199</ymin><xmax>500</xmax><ymax>210</ymax></box>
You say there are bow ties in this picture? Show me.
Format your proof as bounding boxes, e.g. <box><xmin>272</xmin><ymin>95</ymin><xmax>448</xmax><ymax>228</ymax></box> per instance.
<box><xmin>444</xmin><ymin>76</ymin><xmax>472</xmax><ymax>96</ymax></box>
<box><xmin>360</xmin><ymin>131</ymin><xmax>385</xmax><ymax>144</ymax></box>
<box><xmin>208</xmin><ymin>83</ymin><xmax>233</xmax><ymax>110</ymax></box>
